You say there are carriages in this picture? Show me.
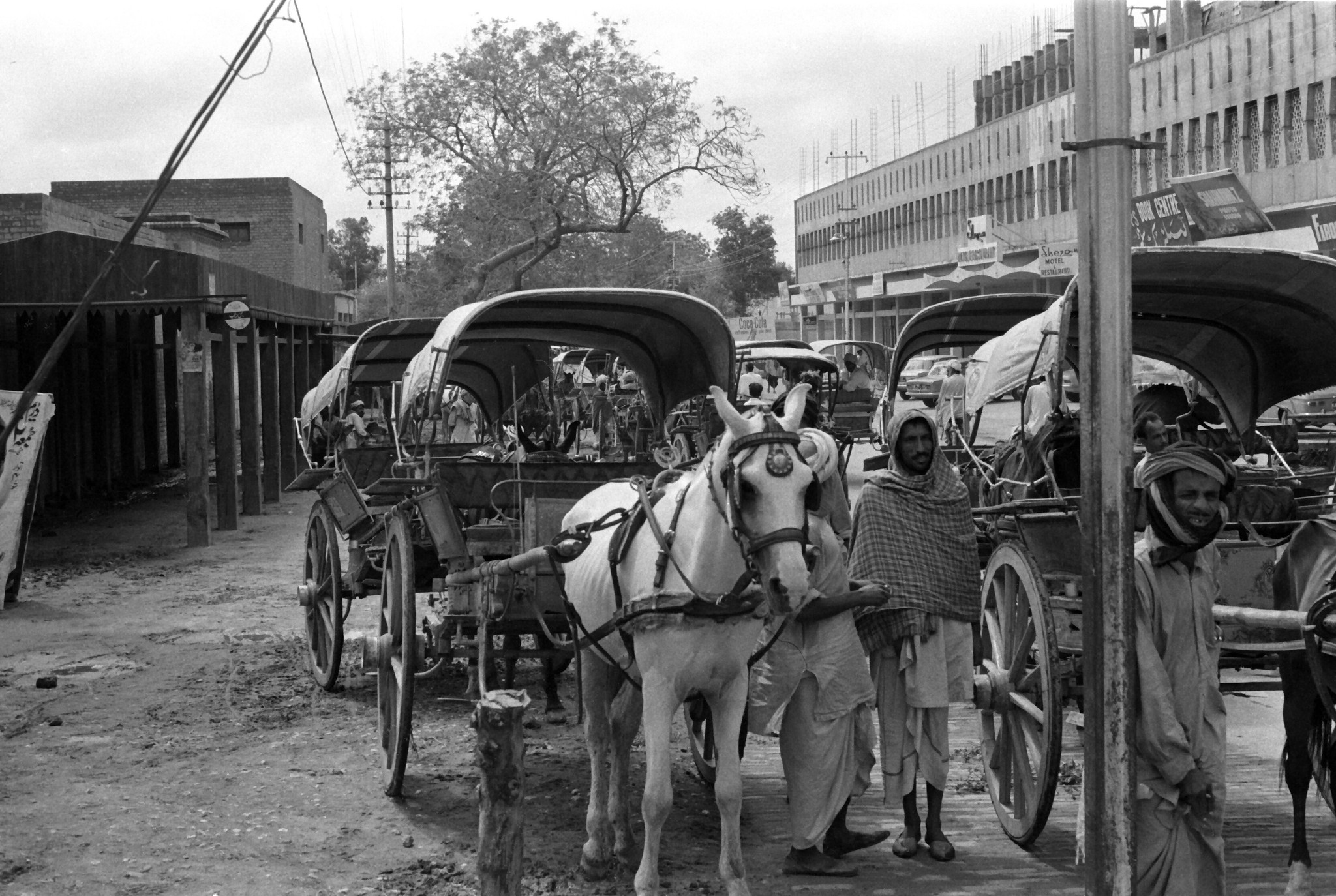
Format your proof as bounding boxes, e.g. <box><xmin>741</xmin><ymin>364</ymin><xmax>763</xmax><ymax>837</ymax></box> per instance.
<box><xmin>295</xmin><ymin>282</ymin><xmax>829</xmax><ymax>895</ymax></box>
<box><xmin>742</xmin><ymin>241</ymin><xmax>1336</xmax><ymax>893</ymax></box>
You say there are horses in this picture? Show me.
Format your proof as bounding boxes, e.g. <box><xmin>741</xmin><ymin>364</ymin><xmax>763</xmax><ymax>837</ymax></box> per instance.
<box><xmin>560</xmin><ymin>384</ymin><xmax>814</xmax><ymax>896</ymax></box>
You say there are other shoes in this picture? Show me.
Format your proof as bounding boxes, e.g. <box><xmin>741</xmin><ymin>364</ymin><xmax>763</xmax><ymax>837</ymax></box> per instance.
<box><xmin>925</xmin><ymin>831</ymin><xmax>955</xmax><ymax>862</ymax></box>
<box><xmin>782</xmin><ymin>853</ymin><xmax>858</xmax><ymax>877</ymax></box>
<box><xmin>823</xmin><ymin>830</ymin><xmax>890</xmax><ymax>857</ymax></box>
<box><xmin>892</xmin><ymin>832</ymin><xmax>922</xmax><ymax>858</ymax></box>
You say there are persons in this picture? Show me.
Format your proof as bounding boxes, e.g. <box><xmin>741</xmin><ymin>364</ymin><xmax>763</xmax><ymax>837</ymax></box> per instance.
<box><xmin>341</xmin><ymin>400</ymin><xmax>372</xmax><ymax>450</ymax></box>
<box><xmin>856</xmin><ymin>348</ymin><xmax>870</xmax><ymax>376</ymax></box>
<box><xmin>1133</xmin><ymin>442</ymin><xmax>1235</xmax><ymax>895</ymax></box>
<box><xmin>734</xmin><ymin>356</ymin><xmax>784</xmax><ymax>407</ymax></box>
<box><xmin>735</xmin><ymin>424</ymin><xmax>898</xmax><ymax>878</ymax></box>
<box><xmin>592</xmin><ymin>373</ymin><xmax>623</xmax><ymax>454</ymax></box>
<box><xmin>1024</xmin><ymin>375</ymin><xmax>1073</xmax><ymax>435</ymax></box>
<box><xmin>1134</xmin><ymin>409</ymin><xmax>1173</xmax><ymax>531</ymax></box>
<box><xmin>933</xmin><ymin>358</ymin><xmax>974</xmax><ymax>447</ymax></box>
<box><xmin>839</xmin><ymin>404</ymin><xmax>980</xmax><ymax>863</ymax></box>
<box><xmin>448</xmin><ymin>388</ymin><xmax>479</xmax><ymax>444</ymax></box>
<box><xmin>831</xmin><ymin>352</ymin><xmax>874</xmax><ymax>410</ymax></box>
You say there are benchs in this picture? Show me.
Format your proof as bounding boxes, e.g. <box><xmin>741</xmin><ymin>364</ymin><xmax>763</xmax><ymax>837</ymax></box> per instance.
<box><xmin>1216</xmin><ymin>483</ymin><xmax>1302</xmax><ymax>652</ymax></box>
<box><xmin>434</xmin><ymin>459</ymin><xmax>670</xmax><ymax>562</ymax></box>
<box><xmin>1167</xmin><ymin>421</ymin><xmax>1336</xmax><ymax>494</ymax></box>
<box><xmin>338</xmin><ymin>440</ymin><xmax>488</xmax><ymax>519</ymax></box>
